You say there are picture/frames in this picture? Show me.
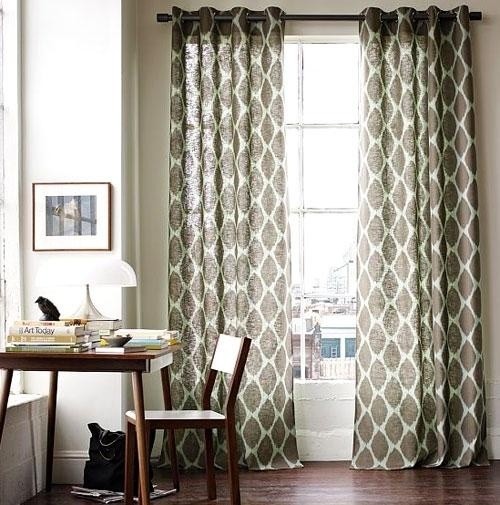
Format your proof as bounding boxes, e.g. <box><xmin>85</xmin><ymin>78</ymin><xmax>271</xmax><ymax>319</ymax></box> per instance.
<box><xmin>33</xmin><ymin>182</ymin><xmax>112</xmax><ymax>252</ymax></box>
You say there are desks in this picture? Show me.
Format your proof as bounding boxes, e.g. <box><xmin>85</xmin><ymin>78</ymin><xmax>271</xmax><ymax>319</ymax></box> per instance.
<box><xmin>0</xmin><ymin>341</ymin><xmax>185</xmax><ymax>505</ymax></box>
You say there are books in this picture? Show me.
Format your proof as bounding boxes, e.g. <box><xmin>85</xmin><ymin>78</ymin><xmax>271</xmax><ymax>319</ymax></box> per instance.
<box><xmin>3</xmin><ymin>317</ymin><xmax>184</xmax><ymax>354</ymax></box>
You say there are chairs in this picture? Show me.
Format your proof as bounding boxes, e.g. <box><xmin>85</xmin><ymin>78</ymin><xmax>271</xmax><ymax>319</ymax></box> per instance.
<box><xmin>122</xmin><ymin>332</ymin><xmax>252</xmax><ymax>505</ymax></box>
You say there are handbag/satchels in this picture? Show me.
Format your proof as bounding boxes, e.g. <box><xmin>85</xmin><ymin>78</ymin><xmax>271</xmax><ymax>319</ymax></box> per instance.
<box><xmin>83</xmin><ymin>420</ymin><xmax>156</xmax><ymax>494</ymax></box>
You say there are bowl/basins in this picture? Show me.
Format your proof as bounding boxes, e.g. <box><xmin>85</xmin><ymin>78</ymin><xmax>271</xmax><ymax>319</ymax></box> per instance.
<box><xmin>102</xmin><ymin>335</ymin><xmax>132</xmax><ymax>347</ymax></box>
